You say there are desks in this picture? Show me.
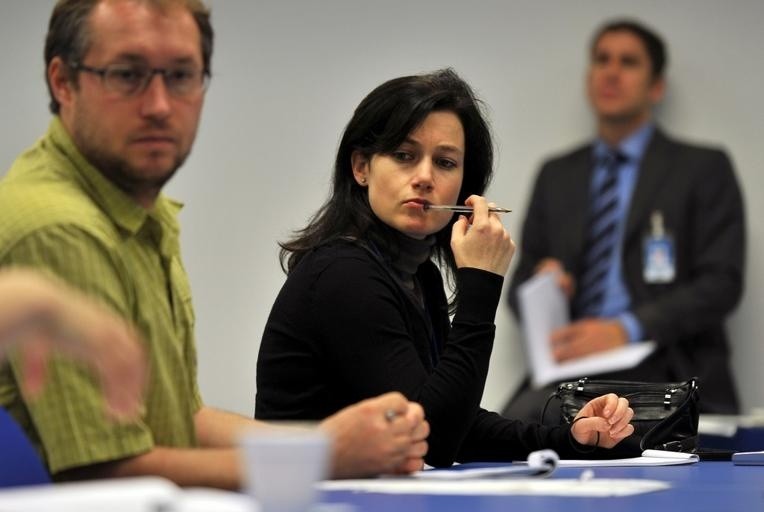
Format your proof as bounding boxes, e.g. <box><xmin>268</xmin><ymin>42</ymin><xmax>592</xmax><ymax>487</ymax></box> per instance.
<box><xmin>226</xmin><ymin>453</ymin><xmax>763</xmax><ymax>511</ymax></box>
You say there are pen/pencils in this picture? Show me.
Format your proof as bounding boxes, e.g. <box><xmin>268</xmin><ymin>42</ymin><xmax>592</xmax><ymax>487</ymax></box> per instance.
<box><xmin>386</xmin><ymin>411</ymin><xmax>394</xmax><ymax>420</ymax></box>
<box><xmin>424</xmin><ymin>205</ymin><xmax>512</xmax><ymax>213</ymax></box>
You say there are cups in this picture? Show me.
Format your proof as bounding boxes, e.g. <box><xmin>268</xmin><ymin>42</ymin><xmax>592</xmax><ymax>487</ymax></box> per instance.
<box><xmin>239</xmin><ymin>428</ymin><xmax>330</xmax><ymax>511</ymax></box>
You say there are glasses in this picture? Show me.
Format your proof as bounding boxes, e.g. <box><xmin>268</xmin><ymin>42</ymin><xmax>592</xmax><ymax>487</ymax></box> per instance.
<box><xmin>70</xmin><ymin>60</ymin><xmax>212</xmax><ymax>100</ymax></box>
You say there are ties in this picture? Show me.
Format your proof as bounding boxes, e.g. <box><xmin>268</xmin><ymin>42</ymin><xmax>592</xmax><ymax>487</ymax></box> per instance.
<box><xmin>573</xmin><ymin>148</ymin><xmax>624</xmax><ymax>319</ymax></box>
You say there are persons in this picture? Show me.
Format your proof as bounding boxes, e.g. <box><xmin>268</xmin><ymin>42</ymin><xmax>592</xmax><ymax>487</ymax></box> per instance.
<box><xmin>1</xmin><ymin>0</ymin><xmax>430</xmax><ymax>491</ymax></box>
<box><xmin>0</xmin><ymin>264</ymin><xmax>147</xmax><ymax>490</ymax></box>
<box><xmin>508</xmin><ymin>19</ymin><xmax>745</xmax><ymax>465</ymax></box>
<box><xmin>254</xmin><ymin>70</ymin><xmax>633</xmax><ymax>469</ymax></box>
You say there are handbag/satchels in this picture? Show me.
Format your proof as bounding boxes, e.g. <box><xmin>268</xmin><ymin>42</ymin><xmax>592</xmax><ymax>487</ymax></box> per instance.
<box><xmin>539</xmin><ymin>376</ymin><xmax>703</xmax><ymax>459</ymax></box>
